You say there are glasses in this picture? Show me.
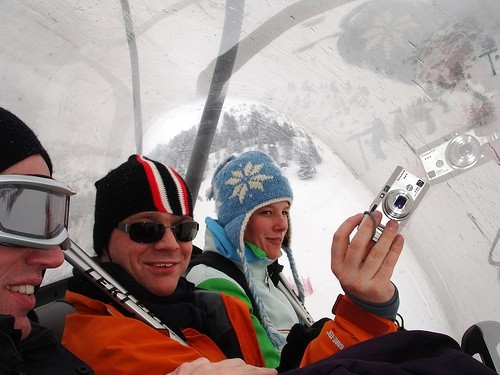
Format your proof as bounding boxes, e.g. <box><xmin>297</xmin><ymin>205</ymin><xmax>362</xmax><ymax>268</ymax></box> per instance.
<box><xmin>0</xmin><ymin>174</ymin><xmax>76</xmax><ymax>251</ymax></box>
<box><xmin>118</xmin><ymin>221</ymin><xmax>200</xmax><ymax>245</ymax></box>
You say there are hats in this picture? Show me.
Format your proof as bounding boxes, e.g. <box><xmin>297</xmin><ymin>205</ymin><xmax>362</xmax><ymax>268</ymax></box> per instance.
<box><xmin>93</xmin><ymin>154</ymin><xmax>193</xmax><ymax>256</ymax></box>
<box><xmin>0</xmin><ymin>107</ymin><xmax>53</xmax><ymax>176</ymax></box>
<box><xmin>206</xmin><ymin>151</ymin><xmax>305</xmax><ymax>349</ymax></box>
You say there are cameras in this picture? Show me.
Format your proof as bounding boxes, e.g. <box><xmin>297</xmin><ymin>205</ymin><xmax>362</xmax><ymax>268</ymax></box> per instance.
<box><xmin>357</xmin><ymin>165</ymin><xmax>430</xmax><ymax>243</ymax></box>
<box><xmin>416</xmin><ymin>108</ymin><xmax>500</xmax><ymax>186</ymax></box>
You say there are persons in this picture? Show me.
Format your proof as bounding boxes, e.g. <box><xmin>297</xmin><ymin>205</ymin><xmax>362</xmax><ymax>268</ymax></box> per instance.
<box><xmin>63</xmin><ymin>153</ymin><xmax>405</xmax><ymax>375</ymax></box>
<box><xmin>203</xmin><ymin>151</ymin><xmax>314</xmax><ymax>353</ymax></box>
<box><xmin>0</xmin><ymin>105</ymin><xmax>500</xmax><ymax>375</ymax></box>
